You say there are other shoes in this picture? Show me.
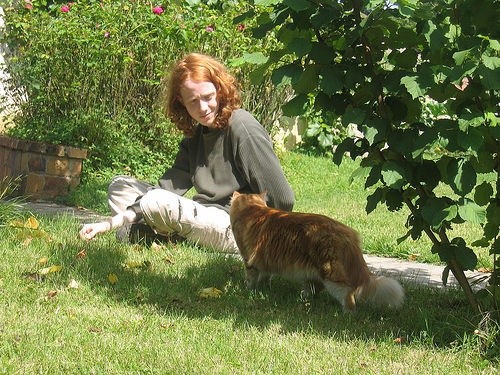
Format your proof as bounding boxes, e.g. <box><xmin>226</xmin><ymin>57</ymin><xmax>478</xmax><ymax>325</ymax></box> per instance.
<box><xmin>129</xmin><ymin>223</ymin><xmax>168</xmax><ymax>249</ymax></box>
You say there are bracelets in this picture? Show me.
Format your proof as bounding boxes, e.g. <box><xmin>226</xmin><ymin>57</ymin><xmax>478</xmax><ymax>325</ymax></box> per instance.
<box><xmin>104</xmin><ymin>216</ymin><xmax>114</xmax><ymax>231</ymax></box>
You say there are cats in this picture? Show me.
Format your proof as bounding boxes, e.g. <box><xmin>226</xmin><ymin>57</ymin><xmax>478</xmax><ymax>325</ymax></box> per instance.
<box><xmin>229</xmin><ymin>191</ymin><xmax>405</xmax><ymax>315</ymax></box>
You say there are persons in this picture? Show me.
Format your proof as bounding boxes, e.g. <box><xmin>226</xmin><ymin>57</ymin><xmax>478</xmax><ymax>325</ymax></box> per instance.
<box><xmin>78</xmin><ymin>52</ymin><xmax>296</xmax><ymax>254</ymax></box>
